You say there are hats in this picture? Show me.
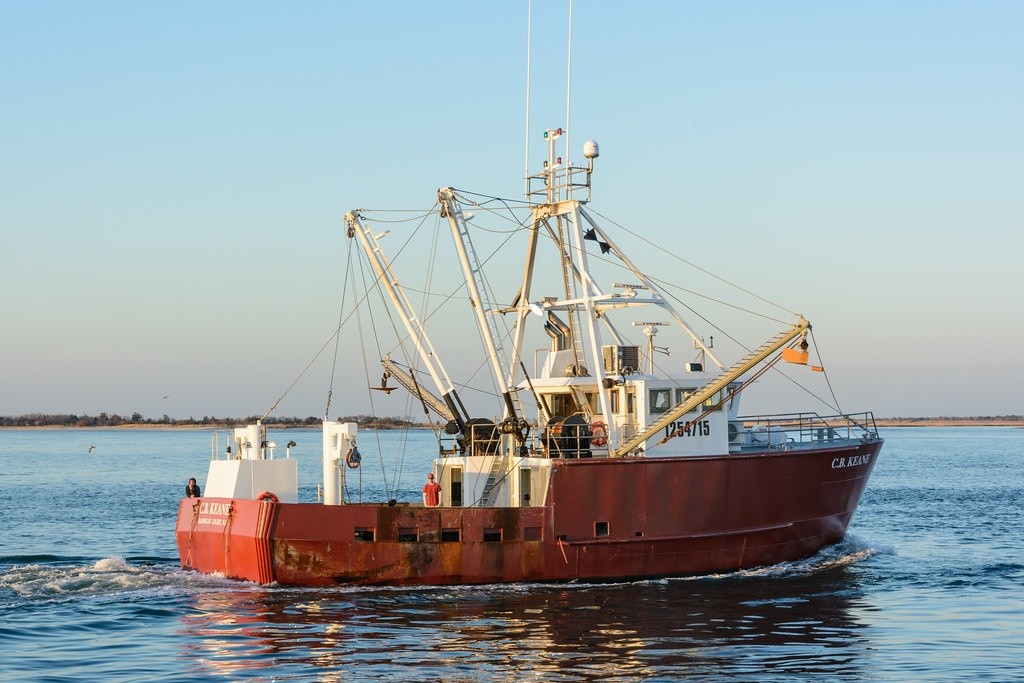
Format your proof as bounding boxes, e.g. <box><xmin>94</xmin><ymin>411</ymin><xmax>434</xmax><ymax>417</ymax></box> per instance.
<box><xmin>428</xmin><ymin>473</ymin><xmax>433</xmax><ymax>478</ymax></box>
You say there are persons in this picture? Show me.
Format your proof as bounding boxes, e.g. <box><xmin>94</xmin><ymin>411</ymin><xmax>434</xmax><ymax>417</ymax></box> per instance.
<box><xmin>422</xmin><ymin>473</ymin><xmax>443</xmax><ymax>507</ymax></box>
<box><xmin>185</xmin><ymin>478</ymin><xmax>201</xmax><ymax>498</ymax></box>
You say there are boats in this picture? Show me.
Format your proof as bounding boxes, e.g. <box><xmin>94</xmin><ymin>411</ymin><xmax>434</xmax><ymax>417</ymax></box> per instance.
<box><xmin>175</xmin><ymin>2</ymin><xmax>886</xmax><ymax>595</ymax></box>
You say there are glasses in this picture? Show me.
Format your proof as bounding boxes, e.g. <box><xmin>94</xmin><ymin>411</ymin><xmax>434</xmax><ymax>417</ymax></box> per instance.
<box><xmin>429</xmin><ymin>478</ymin><xmax>433</xmax><ymax>479</ymax></box>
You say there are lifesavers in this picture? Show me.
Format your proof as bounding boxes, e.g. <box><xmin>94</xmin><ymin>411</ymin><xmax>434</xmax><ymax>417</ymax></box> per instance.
<box><xmin>589</xmin><ymin>421</ymin><xmax>607</xmax><ymax>447</ymax></box>
